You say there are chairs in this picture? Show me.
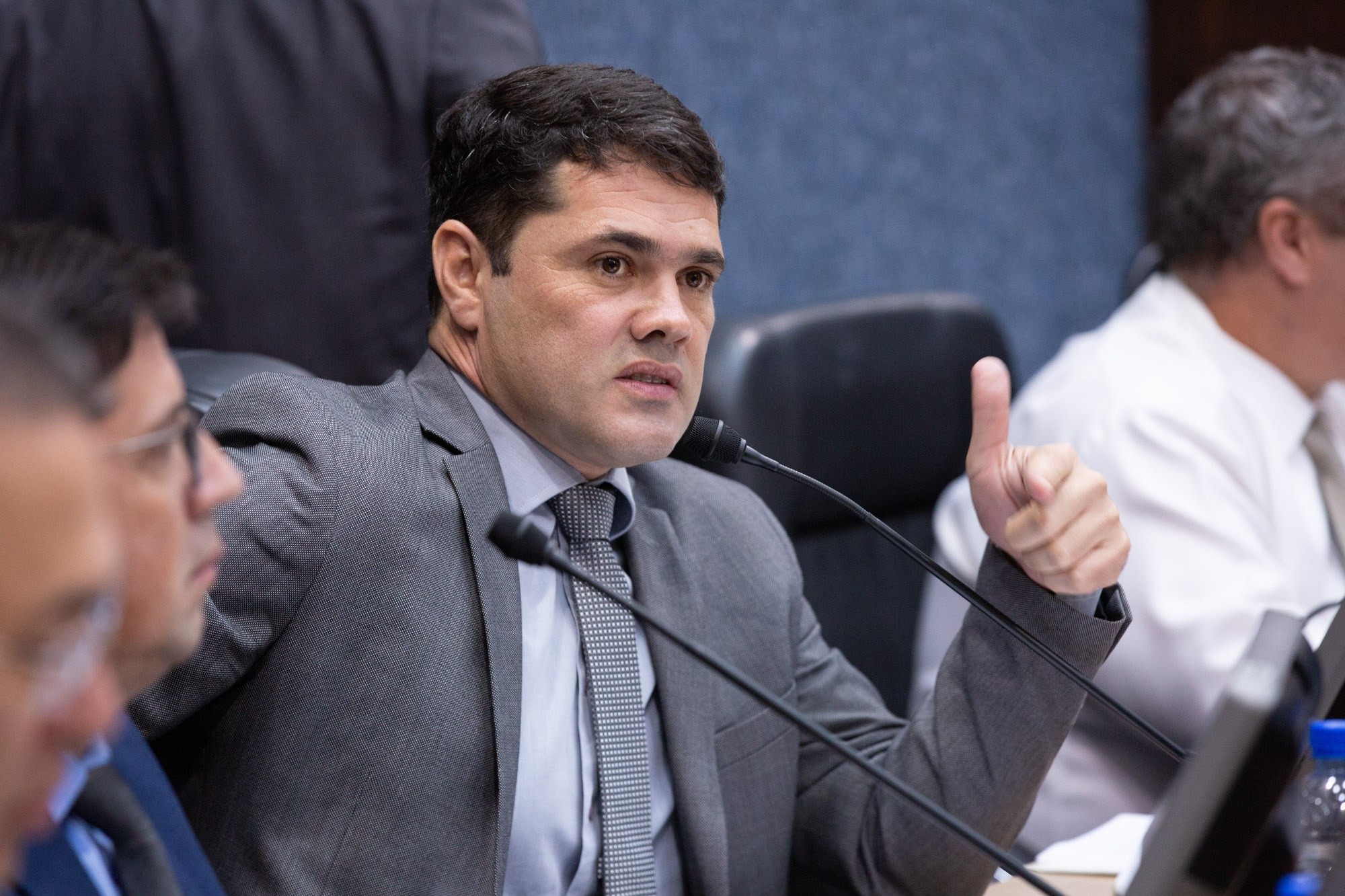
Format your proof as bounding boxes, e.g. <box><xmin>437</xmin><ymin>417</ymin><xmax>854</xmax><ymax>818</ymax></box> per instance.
<box><xmin>698</xmin><ymin>289</ymin><xmax>1019</xmax><ymax>722</ymax></box>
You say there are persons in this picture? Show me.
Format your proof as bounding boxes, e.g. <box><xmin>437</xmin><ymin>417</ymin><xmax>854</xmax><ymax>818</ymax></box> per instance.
<box><xmin>126</xmin><ymin>64</ymin><xmax>1132</xmax><ymax>896</ymax></box>
<box><xmin>0</xmin><ymin>216</ymin><xmax>244</xmax><ymax>896</ymax></box>
<box><xmin>1</xmin><ymin>0</ymin><xmax>546</xmax><ymax>387</ymax></box>
<box><xmin>912</xmin><ymin>47</ymin><xmax>1344</xmax><ymax>880</ymax></box>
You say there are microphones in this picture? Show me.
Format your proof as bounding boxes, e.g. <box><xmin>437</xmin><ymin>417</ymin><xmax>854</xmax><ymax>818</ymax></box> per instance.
<box><xmin>683</xmin><ymin>413</ymin><xmax>1189</xmax><ymax>764</ymax></box>
<box><xmin>484</xmin><ymin>510</ymin><xmax>1069</xmax><ymax>896</ymax></box>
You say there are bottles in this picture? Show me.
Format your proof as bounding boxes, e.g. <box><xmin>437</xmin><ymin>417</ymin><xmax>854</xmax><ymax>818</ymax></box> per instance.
<box><xmin>1273</xmin><ymin>719</ymin><xmax>1344</xmax><ymax>896</ymax></box>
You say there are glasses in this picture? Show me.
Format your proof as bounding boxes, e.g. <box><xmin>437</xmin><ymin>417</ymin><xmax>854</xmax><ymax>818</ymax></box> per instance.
<box><xmin>106</xmin><ymin>388</ymin><xmax>221</xmax><ymax>480</ymax></box>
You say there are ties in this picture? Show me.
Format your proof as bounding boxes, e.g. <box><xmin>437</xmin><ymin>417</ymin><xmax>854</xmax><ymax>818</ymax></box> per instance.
<box><xmin>71</xmin><ymin>766</ymin><xmax>177</xmax><ymax>895</ymax></box>
<box><xmin>547</xmin><ymin>483</ymin><xmax>657</xmax><ymax>895</ymax></box>
<box><xmin>1302</xmin><ymin>410</ymin><xmax>1342</xmax><ymax>550</ymax></box>
